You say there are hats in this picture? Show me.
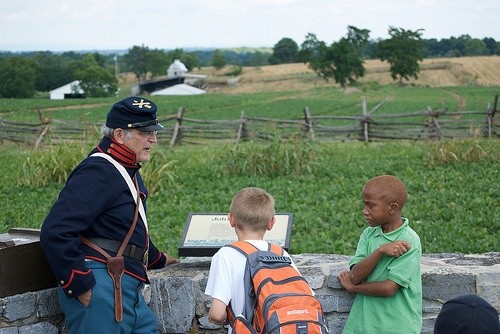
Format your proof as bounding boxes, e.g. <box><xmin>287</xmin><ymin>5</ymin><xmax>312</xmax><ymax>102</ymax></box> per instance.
<box><xmin>105</xmin><ymin>97</ymin><xmax>165</xmax><ymax>132</ymax></box>
<box><xmin>434</xmin><ymin>295</ymin><xmax>500</xmax><ymax>334</ymax></box>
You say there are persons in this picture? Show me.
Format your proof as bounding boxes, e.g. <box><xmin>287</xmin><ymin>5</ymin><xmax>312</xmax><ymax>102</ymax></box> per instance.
<box><xmin>338</xmin><ymin>175</ymin><xmax>423</xmax><ymax>334</ymax></box>
<box><xmin>434</xmin><ymin>296</ymin><xmax>500</xmax><ymax>334</ymax></box>
<box><xmin>203</xmin><ymin>186</ymin><xmax>316</xmax><ymax>334</ymax></box>
<box><xmin>39</xmin><ymin>96</ymin><xmax>178</xmax><ymax>334</ymax></box>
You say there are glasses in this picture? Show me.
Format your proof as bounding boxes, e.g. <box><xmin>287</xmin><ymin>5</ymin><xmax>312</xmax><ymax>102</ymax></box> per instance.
<box><xmin>136</xmin><ymin>131</ymin><xmax>158</xmax><ymax>138</ymax></box>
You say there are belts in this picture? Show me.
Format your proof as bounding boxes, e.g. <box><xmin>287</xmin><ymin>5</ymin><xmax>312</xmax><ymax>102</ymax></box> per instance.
<box><xmin>82</xmin><ymin>236</ymin><xmax>149</xmax><ymax>263</ymax></box>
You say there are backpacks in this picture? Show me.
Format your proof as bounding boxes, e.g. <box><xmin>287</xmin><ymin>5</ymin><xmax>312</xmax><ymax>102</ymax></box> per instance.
<box><xmin>221</xmin><ymin>240</ymin><xmax>329</xmax><ymax>334</ymax></box>
